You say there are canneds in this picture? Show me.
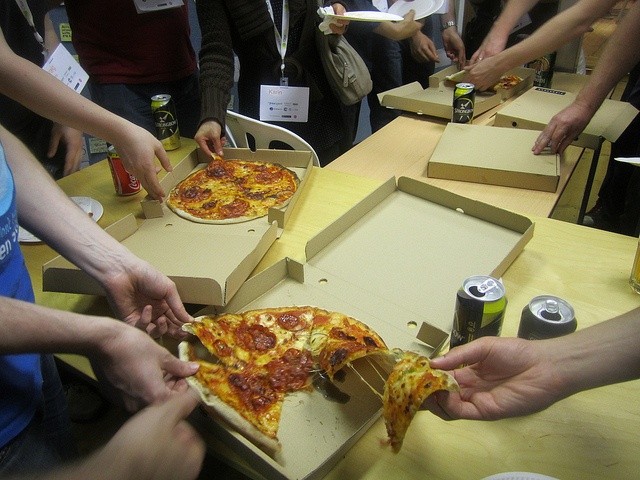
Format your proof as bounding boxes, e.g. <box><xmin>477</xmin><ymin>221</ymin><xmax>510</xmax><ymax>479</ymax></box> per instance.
<box><xmin>451</xmin><ymin>276</ymin><xmax>507</xmax><ymax>352</ymax></box>
<box><xmin>151</xmin><ymin>94</ymin><xmax>180</xmax><ymax>151</ymax></box>
<box><xmin>107</xmin><ymin>145</ymin><xmax>143</xmax><ymax>196</ymax></box>
<box><xmin>524</xmin><ymin>51</ymin><xmax>557</xmax><ymax>88</ymax></box>
<box><xmin>452</xmin><ymin>83</ymin><xmax>475</xmax><ymax>124</ymax></box>
<box><xmin>517</xmin><ymin>294</ymin><xmax>577</xmax><ymax>340</ymax></box>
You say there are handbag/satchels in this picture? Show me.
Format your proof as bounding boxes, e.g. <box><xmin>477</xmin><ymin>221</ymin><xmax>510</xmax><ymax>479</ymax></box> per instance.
<box><xmin>316</xmin><ymin>28</ymin><xmax>373</xmax><ymax>105</ymax></box>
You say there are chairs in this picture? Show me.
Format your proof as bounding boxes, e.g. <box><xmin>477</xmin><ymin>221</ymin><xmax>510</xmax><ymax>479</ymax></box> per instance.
<box><xmin>223</xmin><ymin>109</ymin><xmax>321</xmax><ymax>166</ymax></box>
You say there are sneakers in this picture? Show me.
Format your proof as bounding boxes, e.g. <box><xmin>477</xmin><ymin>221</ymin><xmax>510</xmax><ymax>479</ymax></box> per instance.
<box><xmin>582</xmin><ymin>198</ymin><xmax>617</xmax><ymax>227</ymax></box>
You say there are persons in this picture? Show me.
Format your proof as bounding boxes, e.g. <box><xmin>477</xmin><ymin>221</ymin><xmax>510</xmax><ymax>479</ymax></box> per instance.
<box><xmin>0</xmin><ymin>121</ymin><xmax>199</xmax><ymax>480</ymax></box>
<box><xmin>465</xmin><ymin>0</ymin><xmax>640</xmax><ymax>229</ymax></box>
<box><xmin>530</xmin><ymin>0</ymin><xmax>640</xmax><ymax>157</ymax></box>
<box><xmin>1</xmin><ymin>0</ymin><xmax>173</xmax><ymax>203</ymax></box>
<box><xmin>194</xmin><ymin>0</ymin><xmax>366</xmax><ymax>169</ymax></box>
<box><xmin>64</xmin><ymin>0</ymin><xmax>204</xmax><ymax>141</ymax></box>
<box><xmin>418</xmin><ymin>306</ymin><xmax>640</xmax><ymax>422</ymax></box>
<box><xmin>345</xmin><ymin>0</ymin><xmax>467</xmax><ymax>135</ymax></box>
<box><xmin>47</xmin><ymin>387</ymin><xmax>207</xmax><ymax>478</ymax></box>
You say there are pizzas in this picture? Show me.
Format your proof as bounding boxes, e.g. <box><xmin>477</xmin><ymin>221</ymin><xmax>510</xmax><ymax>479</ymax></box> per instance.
<box><xmin>385</xmin><ymin>350</ymin><xmax>460</xmax><ymax>453</ymax></box>
<box><xmin>167</xmin><ymin>153</ymin><xmax>302</xmax><ymax>224</ymax></box>
<box><xmin>179</xmin><ymin>307</ymin><xmax>393</xmax><ymax>467</ymax></box>
<box><xmin>444</xmin><ymin>69</ymin><xmax>523</xmax><ymax>91</ymax></box>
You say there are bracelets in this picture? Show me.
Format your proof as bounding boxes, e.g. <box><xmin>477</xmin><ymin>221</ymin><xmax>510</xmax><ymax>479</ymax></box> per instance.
<box><xmin>439</xmin><ymin>20</ymin><xmax>456</xmax><ymax>31</ymax></box>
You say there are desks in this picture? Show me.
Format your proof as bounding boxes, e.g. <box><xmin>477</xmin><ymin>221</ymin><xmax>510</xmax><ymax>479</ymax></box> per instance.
<box><xmin>19</xmin><ymin>134</ymin><xmax>640</xmax><ymax>479</ymax></box>
<box><xmin>322</xmin><ymin>72</ymin><xmax>616</xmax><ymax>219</ymax></box>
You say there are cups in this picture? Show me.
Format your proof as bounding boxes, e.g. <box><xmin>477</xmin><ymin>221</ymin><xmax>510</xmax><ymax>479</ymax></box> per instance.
<box><xmin>628</xmin><ymin>237</ymin><xmax>640</xmax><ymax>294</ymax></box>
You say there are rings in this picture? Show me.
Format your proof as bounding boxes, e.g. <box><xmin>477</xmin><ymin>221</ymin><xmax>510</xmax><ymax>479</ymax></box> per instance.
<box><xmin>475</xmin><ymin>57</ymin><xmax>483</xmax><ymax>61</ymax></box>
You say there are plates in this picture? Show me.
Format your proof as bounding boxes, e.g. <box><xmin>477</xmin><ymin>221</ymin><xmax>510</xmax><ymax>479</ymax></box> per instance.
<box><xmin>18</xmin><ymin>197</ymin><xmax>104</xmax><ymax>244</ymax></box>
<box><xmin>388</xmin><ymin>0</ymin><xmax>444</xmax><ymax>20</ymax></box>
<box><xmin>614</xmin><ymin>157</ymin><xmax>640</xmax><ymax>167</ymax></box>
<box><xmin>325</xmin><ymin>11</ymin><xmax>404</xmax><ymax>22</ymax></box>
